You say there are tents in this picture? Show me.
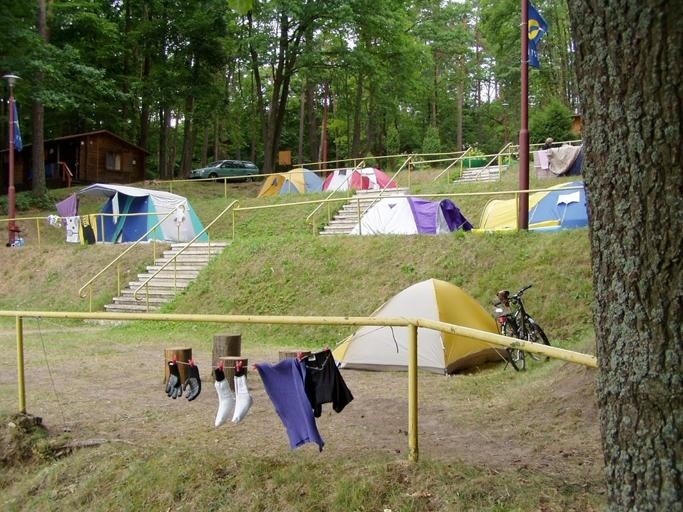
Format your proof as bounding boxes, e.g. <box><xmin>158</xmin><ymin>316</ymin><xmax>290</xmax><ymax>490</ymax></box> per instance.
<box><xmin>480</xmin><ymin>181</ymin><xmax>588</xmax><ymax>231</ymax></box>
<box><xmin>332</xmin><ymin>278</ymin><xmax>509</xmax><ymax>374</ymax></box>
<box><xmin>323</xmin><ymin>168</ymin><xmax>398</xmax><ymax>192</ymax></box>
<box><xmin>80</xmin><ymin>185</ymin><xmax>208</xmax><ymax>243</ymax></box>
<box><xmin>350</xmin><ymin>198</ymin><xmax>473</xmax><ymax>235</ymax></box>
<box><xmin>258</xmin><ymin>168</ymin><xmax>322</xmax><ymax>197</ymax></box>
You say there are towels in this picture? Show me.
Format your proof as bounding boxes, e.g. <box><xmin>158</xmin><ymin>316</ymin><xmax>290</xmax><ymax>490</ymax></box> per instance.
<box><xmin>254</xmin><ymin>358</ymin><xmax>325</xmax><ymax>453</ymax></box>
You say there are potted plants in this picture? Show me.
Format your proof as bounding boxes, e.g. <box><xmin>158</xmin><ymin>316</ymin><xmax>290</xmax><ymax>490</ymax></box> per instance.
<box><xmin>463</xmin><ymin>150</ymin><xmax>485</xmax><ymax>167</ymax></box>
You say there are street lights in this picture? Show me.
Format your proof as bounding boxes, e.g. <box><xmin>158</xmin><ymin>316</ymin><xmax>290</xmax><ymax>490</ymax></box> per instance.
<box><xmin>502</xmin><ymin>100</ymin><xmax>509</xmax><ymax>146</ymax></box>
<box><xmin>1</xmin><ymin>72</ymin><xmax>24</xmax><ymax>246</ymax></box>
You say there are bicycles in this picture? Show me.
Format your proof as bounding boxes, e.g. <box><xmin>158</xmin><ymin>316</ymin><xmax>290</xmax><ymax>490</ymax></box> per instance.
<box><xmin>489</xmin><ymin>283</ymin><xmax>551</xmax><ymax>372</ymax></box>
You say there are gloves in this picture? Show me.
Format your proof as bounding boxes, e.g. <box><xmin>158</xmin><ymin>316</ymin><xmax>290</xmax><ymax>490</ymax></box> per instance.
<box><xmin>165</xmin><ymin>360</ymin><xmax>182</xmax><ymax>400</ymax></box>
<box><xmin>181</xmin><ymin>364</ymin><xmax>201</xmax><ymax>402</ymax></box>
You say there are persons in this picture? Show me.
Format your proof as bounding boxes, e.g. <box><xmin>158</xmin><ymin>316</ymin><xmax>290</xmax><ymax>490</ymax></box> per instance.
<box><xmin>544</xmin><ymin>138</ymin><xmax>553</xmax><ymax>150</ymax></box>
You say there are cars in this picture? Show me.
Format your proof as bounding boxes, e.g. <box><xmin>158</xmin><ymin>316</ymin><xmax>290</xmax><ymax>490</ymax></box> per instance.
<box><xmin>189</xmin><ymin>159</ymin><xmax>259</xmax><ymax>182</ymax></box>
